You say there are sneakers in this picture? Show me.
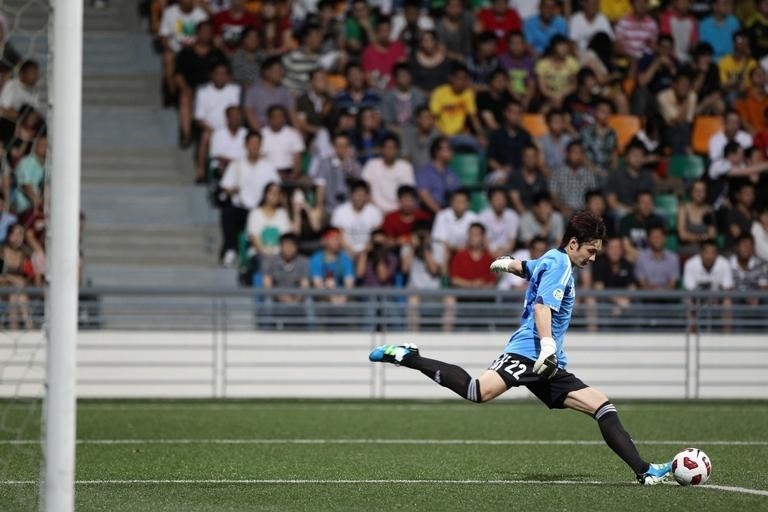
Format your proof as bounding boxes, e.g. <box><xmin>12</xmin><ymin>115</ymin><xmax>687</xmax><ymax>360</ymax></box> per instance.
<box><xmin>636</xmin><ymin>462</ymin><xmax>675</xmax><ymax>486</ymax></box>
<box><xmin>369</xmin><ymin>341</ymin><xmax>419</xmax><ymax>365</ymax></box>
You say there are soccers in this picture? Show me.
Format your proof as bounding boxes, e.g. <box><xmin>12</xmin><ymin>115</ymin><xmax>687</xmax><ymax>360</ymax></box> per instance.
<box><xmin>672</xmin><ymin>448</ymin><xmax>711</xmax><ymax>486</ymax></box>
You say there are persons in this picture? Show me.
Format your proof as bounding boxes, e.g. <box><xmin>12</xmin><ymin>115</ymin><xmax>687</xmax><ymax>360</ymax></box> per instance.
<box><xmin>369</xmin><ymin>212</ymin><xmax>673</xmax><ymax>486</ymax></box>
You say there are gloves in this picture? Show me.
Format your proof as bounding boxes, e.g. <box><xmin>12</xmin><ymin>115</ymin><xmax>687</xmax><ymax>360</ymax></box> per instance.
<box><xmin>532</xmin><ymin>336</ymin><xmax>558</xmax><ymax>379</ymax></box>
<box><xmin>490</xmin><ymin>254</ymin><xmax>515</xmax><ymax>273</ymax></box>
